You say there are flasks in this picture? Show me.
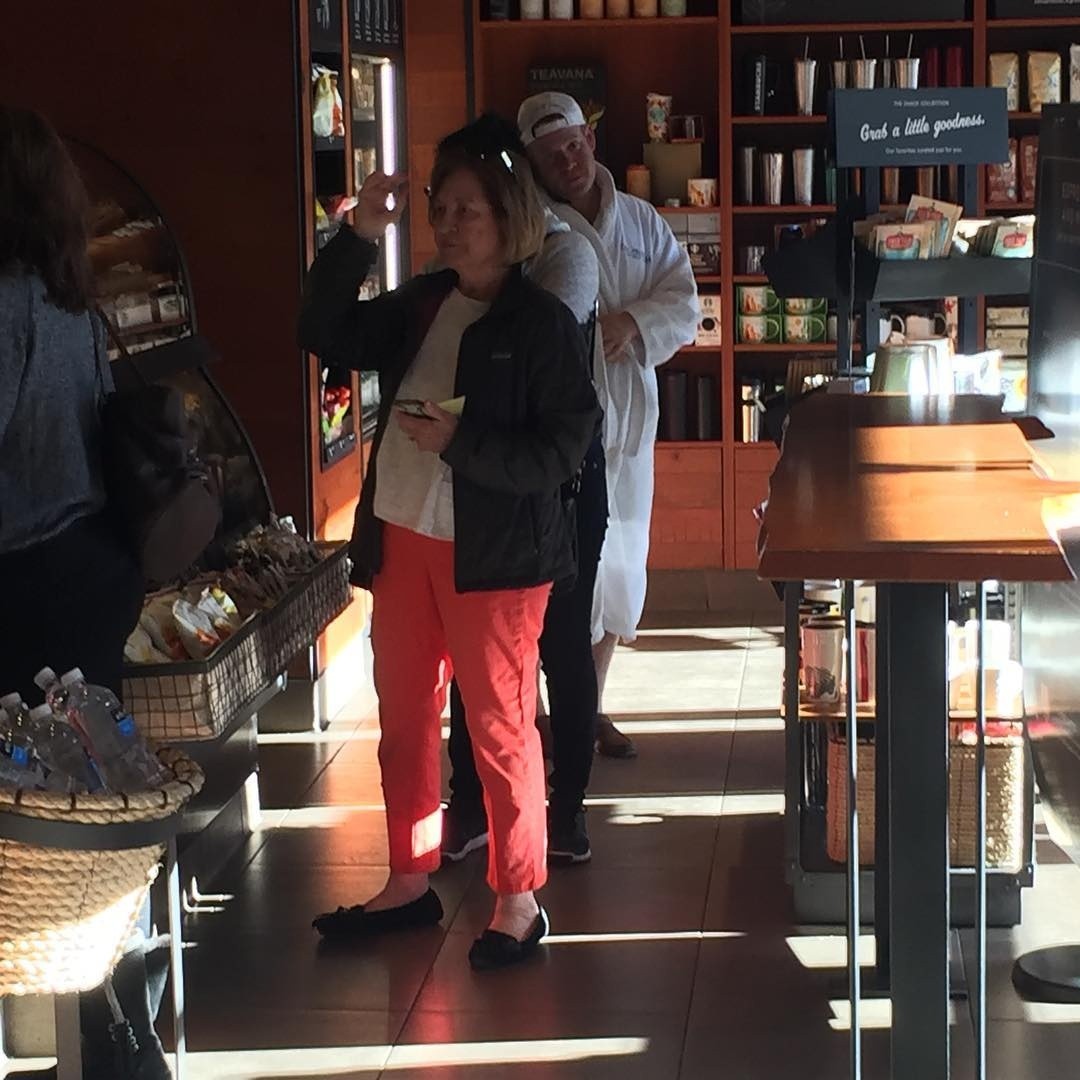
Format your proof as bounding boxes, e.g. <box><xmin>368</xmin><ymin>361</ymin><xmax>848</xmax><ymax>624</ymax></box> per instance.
<box><xmin>741</xmin><ymin>378</ymin><xmax>761</xmax><ymax>443</ymax></box>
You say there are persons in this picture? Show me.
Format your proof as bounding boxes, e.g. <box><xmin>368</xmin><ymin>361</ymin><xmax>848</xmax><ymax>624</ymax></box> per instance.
<box><xmin>295</xmin><ymin>141</ymin><xmax>604</xmax><ymax>968</ymax></box>
<box><xmin>515</xmin><ymin>90</ymin><xmax>699</xmax><ymax>759</ymax></box>
<box><xmin>441</xmin><ymin>116</ymin><xmax>611</xmax><ymax>865</ymax></box>
<box><xmin>0</xmin><ymin>105</ymin><xmax>174</xmax><ymax>1080</ymax></box>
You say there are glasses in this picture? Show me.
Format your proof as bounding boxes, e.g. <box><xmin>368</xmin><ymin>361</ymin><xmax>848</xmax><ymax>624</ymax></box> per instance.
<box><xmin>426</xmin><ymin>195</ymin><xmax>500</xmax><ymax>224</ymax></box>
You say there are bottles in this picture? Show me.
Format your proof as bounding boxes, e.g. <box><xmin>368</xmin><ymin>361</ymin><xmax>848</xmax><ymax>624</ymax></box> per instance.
<box><xmin>627</xmin><ymin>164</ymin><xmax>649</xmax><ymax>201</ymax></box>
<box><xmin>1</xmin><ymin>692</ymin><xmax>45</xmax><ymax>790</ymax></box>
<box><xmin>29</xmin><ymin>666</ymin><xmax>175</xmax><ymax>796</ymax></box>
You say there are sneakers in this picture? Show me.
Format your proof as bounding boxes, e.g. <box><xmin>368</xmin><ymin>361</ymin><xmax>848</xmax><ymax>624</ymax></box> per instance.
<box><xmin>593</xmin><ymin>712</ymin><xmax>637</xmax><ymax>757</ymax></box>
<box><xmin>545</xmin><ymin>806</ymin><xmax>590</xmax><ymax>861</ymax></box>
<box><xmin>535</xmin><ymin>712</ymin><xmax>554</xmax><ymax>753</ymax></box>
<box><xmin>438</xmin><ymin>800</ymin><xmax>487</xmax><ymax>862</ymax></box>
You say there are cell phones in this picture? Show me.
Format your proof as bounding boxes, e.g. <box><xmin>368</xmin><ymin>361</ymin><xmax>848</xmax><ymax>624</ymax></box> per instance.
<box><xmin>393</xmin><ymin>399</ymin><xmax>429</xmax><ymax>419</ymax></box>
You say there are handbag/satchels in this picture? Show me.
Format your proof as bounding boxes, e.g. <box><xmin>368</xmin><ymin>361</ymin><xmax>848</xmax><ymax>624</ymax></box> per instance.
<box><xmin>81</xmin><ymin>304</ymin><xmax>191</xmax><ymax>512</ymax></box>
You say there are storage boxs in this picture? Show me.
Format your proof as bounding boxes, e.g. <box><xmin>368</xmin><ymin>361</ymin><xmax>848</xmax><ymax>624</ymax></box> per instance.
<box><xmin>643</xmin><ymin>144</ymin><xmax>701</xmax><ymax>201</ymax></box>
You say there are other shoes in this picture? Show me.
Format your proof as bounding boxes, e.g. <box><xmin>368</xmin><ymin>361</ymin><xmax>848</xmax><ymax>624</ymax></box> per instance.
<box><xmin>312</xmin><ymin>887</ymin><xmax>443</xmax><ymax>941</ymax></box>
<box><xmin>468</xmin><ymin>906</ymin><xmax>549</xmax><ymax>971</ymax></box>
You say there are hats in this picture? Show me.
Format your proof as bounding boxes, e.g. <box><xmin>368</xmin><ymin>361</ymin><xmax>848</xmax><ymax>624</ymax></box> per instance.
<box><xmin>517</xmin><ymin>93</ymin><xmax>587</xmax><ymax>147</ymax></box>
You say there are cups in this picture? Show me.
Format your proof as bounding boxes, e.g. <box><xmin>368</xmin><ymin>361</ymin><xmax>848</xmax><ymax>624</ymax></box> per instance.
<box><xmin>761</xmin><ymin>148</ymin><xmax>786</xmax><ymax>205</ymax></box>
<box><xmin>795</xmin><ymin>60</ymin><xmax>822</xmax><ymax>116</ymax></box>
<box><xmin>740</xmin><ymin>286</ymin><xmax>779</xmax><ymax>315</ymax></box>
<box><xmin>906</xmin><ymin>312</ymin><xmax>947</xmax><ymax>337</ymax></box>
<box><xmin>792</xmin><ymin>143</ymin><xmax>814</xmax><ymax>205</ymax></box>
<box><xmin>799</xmin><ymin>599</ymin><xmax>837</xmax><ymax>608</ymax></box>
<box><xmin>784</xmin><ymin>315</ymin><xmax>824</xmax><ymax>343</ymax></box>
<box><xmin>854</xmin><ymin>621</ymin><xmax>875</xmax><ymax>701</ymax></box>
<box><xmin>823</xmin><ymin>144</ymin><xmax>837</xmax><ymax>206</ymax></box>
<box><xmin>801</xmin><ymin>623</ymin><xmax>844</xmax><ymax>703</ymax></box>
<box><xmin>808</xmin><ymin>617</ymin><xmax>847</xmax><ymax>625</ymax></box>
<box><xmin>746</xmin><ymin>246</ymin><xmax>765</xmax><ymax>275</ymax></box>
<box><xmin>830</xmin><ymin>58</ymin><xmax>850</xmax><ymax>88</ymax></box>
<box><xmin>646</xmin><ymin>92</ymin><xmax>672</xmax><ymax>143</ymax></box>
<box><xmin>739</xmin><ymin>315</ymin><xmax>779</xmax><ymax>343</ymax></box>
<box><xmin>786</xmin><ymin>298</ymin><xmax>825</xmax><ymax>314</ymax></box>
<box><xmin>877</xmin><ymin>57</ymin><xmax>893</xmax><ymax>89</ymax></box>
<box><xmin>893</xmin><ymin>58</ymin><xmax>920</xmax><ymax>89</ymax></box>
<box><xmin>688</xmin><ymin>179</ymin><xmax>718</xmax><ymax>206</ymax></box>
<box><xmin>799</xmin><ymin>608</ymin><xmax>834</xmax><ymax>617</ymax></box>
<box><xmin>853</xmin><ymin>59</ymin><xmax>877</xmax><ymax>89</ymax></box>
<box><xmin>879</xmin><ymin>314</ymin><xmax>905</xmax><ymax>343</ymax></box>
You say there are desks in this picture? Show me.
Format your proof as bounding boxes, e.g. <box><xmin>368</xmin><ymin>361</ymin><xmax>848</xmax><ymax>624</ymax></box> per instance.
<box><xmin>757</xmin><ymin>393</ymin><xmax>1077</xmax><ymax>1080</ymax></box>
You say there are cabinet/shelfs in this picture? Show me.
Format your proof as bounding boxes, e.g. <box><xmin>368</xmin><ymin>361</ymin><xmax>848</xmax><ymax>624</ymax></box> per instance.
<box><xmin>0</xmin><ymin>0</ymin><xmax>1080</xmax><ymax>731</ymax></box>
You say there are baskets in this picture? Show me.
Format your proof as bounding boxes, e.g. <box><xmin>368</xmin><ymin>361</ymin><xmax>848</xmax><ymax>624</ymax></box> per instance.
<box><xmin>0</xmin><ymin>744</ymin><xmax>203</xmax><ymax>997</ymax></box>
<box><xmin>124</xmin><ymin>540</ymin><xmax>354</xmax><ymax>742</ymax></box>
<box><xmin>828</xmin><ymin>733</ymin><xmax>1017</xmax><ymax>868</ymax></box>
